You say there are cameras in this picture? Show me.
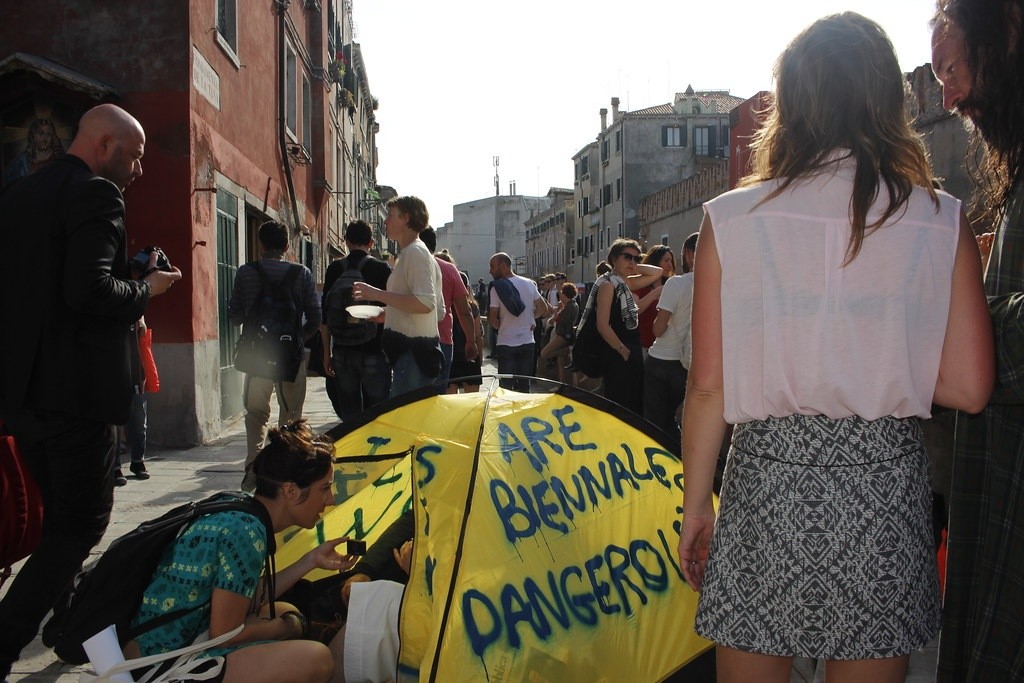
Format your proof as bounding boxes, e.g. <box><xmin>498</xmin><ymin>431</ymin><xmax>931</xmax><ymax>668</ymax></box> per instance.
<box><xmin>128</xmin><ymin>245</ymin><xmax>173</xmax><ymax>278</ymax></box>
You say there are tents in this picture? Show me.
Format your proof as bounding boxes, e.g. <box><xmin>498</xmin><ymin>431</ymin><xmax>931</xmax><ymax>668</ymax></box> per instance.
<box><xmin>258</xmin><ymin>372</ymin><xmax>722</xmax><ymax>683</ymax></box>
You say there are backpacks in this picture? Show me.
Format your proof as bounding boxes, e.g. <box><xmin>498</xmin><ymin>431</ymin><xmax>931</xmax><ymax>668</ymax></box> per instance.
<box><xmin>41</xmin><ymin>490</ymin><xmax>277</xmax><ymax>666</ymax></box>
<box><xmin>233</xmin><ymin>261</ymin><xmax>303</xmax><ymax>382</ymax></box>
<box><xmin>327</xmin><ymin>256</ymin><xmax>378</xmax><ymax>347</ymax></box>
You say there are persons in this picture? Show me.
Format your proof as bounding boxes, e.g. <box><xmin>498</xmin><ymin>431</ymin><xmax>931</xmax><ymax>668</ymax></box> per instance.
<box><xmin>0</xmin><ymin>102</ymin><xmax>485</xmax><ymax>683</ymax></box>
<box><xmin>487</xmin><ymin>0</ymin><xmax>1024</xmax><ymax>683</ymax></box>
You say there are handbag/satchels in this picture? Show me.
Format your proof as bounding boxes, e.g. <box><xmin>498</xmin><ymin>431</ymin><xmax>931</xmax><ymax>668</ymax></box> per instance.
<box><xmin>141</xmin><ymin>329</ymin><xmax>161</xmax><ymax>393</ymax></box>
<box><xmin>572</xmin><ymin>279</ymin><xmax>617</xmax><ymax>379</ymax></box>
<box><xmin>0</xmin><ymin>435</ymin><xmax>44</xmax><ymax>590</ymax></box>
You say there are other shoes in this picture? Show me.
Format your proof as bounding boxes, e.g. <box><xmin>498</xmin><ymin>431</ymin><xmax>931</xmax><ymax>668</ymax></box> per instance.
<box><xmin>487</xmin><ymin>355</ymin><xmax>497</xmax><ymax>358</ymax></box>
<box><xmin>114</xmin><ymin>469</ymin><xmax>127</xmax><ymax>485</ymax></box>
<box><xmin>130</xmin><ymin>462</ymin><xmax>150</xmax><ymax>478</ymax></box>
<box><xmin>549</xmin><ymin>386</ymin><xmax>559</xmax><ymax>392</ymax></box>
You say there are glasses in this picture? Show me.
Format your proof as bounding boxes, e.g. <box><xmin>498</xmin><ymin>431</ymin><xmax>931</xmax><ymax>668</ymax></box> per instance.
<box><xmin>540</xmin><ymin>283</ymin><xmax>545</xmax><ymax>286</ymax></box>
<box><xmin>554</xmin><ymin>278</ymin><xmax>562</xmax><ymax>280</ymax></box>
<box><xmin>545</xmin><ymin>281</ymin><xmax>552</xmax><ymax>283</ymax></box>
<box><xmin>619</xmin><ymin>252</ymin><xmax>643</xmax><ymax>263</ymax></box>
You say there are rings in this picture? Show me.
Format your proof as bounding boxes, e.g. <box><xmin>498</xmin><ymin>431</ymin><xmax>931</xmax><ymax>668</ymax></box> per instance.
<box><xmin>691</xmin><ymin>561</ymin><xmax>696</xmax><ymax>565</ymax></box>
